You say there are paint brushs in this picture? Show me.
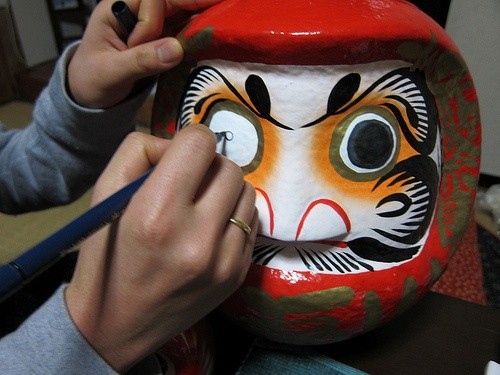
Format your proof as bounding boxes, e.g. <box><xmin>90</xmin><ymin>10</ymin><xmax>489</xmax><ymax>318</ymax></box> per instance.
<box><xmin>0</xmin><ymin>131</ymin><xmax>226</xmax><ymax>300</ymax></box>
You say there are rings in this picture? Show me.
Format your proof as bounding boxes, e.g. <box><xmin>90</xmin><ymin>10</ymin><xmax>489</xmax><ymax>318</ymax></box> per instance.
<box><xmin>229</xmin><ymin>217</ymin><xmax>251</xmax><ymax>235</ymax></box>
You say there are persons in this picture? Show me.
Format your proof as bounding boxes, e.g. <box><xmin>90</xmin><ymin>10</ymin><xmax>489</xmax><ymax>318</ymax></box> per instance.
<box><xmin>0</xmin><ymin>0</ymin><xmax>259</xmax><ymax>375</ymax></box>
